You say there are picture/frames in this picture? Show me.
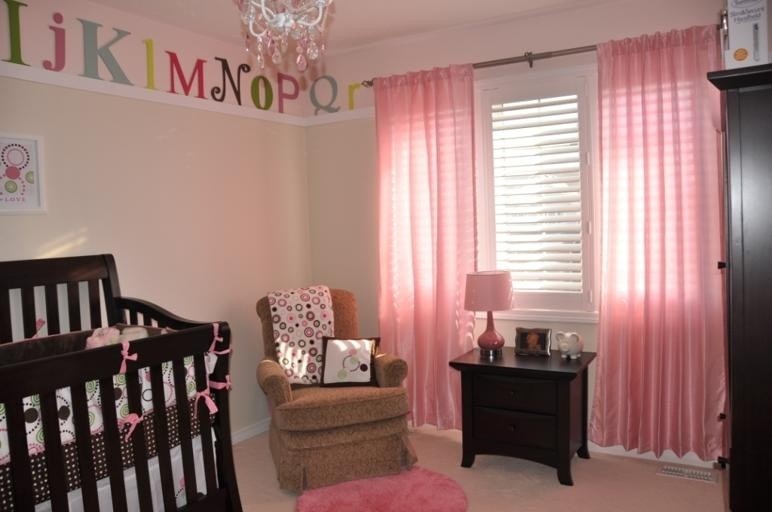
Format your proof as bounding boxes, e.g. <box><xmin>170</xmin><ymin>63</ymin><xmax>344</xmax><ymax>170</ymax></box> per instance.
<box><xmin>514</xmin><ymin>325</ymin><xmax>552</xmax><ymax>357</ymax></box>
<box><xmin>0</xmin><ymin>132</ymin><xmax>47</xmax><ymax>216</ymax></box>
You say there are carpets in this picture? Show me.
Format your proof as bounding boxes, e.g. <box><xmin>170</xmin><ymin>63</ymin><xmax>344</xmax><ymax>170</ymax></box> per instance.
<box><xmin>293</xmin><ymin>466</ymin><xmax>467</xmax><ymax>512</ymax></box>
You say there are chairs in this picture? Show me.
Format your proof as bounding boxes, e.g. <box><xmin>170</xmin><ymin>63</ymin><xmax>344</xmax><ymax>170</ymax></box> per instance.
<box><xmin>254</xmin><ymin>285</ymin><xmax>419</xmax><ymax>495</ymax></box>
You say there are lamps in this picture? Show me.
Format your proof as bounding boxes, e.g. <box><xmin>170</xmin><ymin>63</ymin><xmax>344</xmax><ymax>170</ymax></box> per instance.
<box><xmin>464</xmin><ymin>269</ymin><xmax>513</xmax><ymax>360</ymax></box>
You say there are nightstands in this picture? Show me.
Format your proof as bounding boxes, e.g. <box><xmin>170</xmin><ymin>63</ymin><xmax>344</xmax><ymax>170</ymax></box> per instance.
<box><xmin>448</xmin><ymin>344</ymin><xmax>598</xmax><ymax>486</ymax></box>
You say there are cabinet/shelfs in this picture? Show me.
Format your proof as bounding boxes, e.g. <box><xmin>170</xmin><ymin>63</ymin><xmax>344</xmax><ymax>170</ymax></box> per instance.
<box><xmin>704</xmin><ymin>60</ymin><xmax>772</xmax><ymax>512</ymax></box>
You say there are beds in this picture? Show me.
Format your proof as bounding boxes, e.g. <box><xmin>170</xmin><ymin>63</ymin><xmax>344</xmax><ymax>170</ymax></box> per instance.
<box><xmin>0</xmin><ymin>253</ymin><xmax>243</xmax><ymax>511</ymax></box>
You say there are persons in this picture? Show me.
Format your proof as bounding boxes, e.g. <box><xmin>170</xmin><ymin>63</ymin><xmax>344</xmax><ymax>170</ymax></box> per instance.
<box><xmin>524</xmin><ymin>331</ymin><xmax>543</xmax><ymax>352</ymax></box>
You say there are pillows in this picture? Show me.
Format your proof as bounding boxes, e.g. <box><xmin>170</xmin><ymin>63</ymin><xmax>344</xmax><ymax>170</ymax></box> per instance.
<box><xmin>318</xmin><ymin>336</ymin><xmax>381</xmax><ymax>390</ymax></box>
